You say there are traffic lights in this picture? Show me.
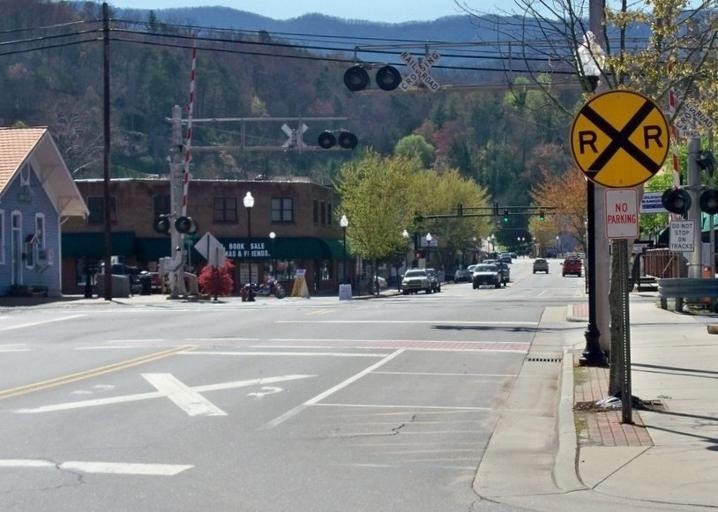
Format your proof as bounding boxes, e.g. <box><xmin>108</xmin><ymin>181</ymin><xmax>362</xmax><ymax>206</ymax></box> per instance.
<box><xmin>415</xmin><ymin>252</ymin><xmax>420</xmax><ymax>261</ymax></box>
<box><xmin>660</xmin><ymin>186</ymin><xmax>718</xmax><ymax>216</ymax></box>
<box><xmin>344</xmin><ymin>65</ymin><xmax>400</xmax><ymax>92</ymax></box>
<box><xmin>319</xmin><ymin>130</ymin><xmax>356</xmax><ymax>149</ymax></box>
<box><xmin>155</xmin><ymin>215</ymin><xmax>196</xmax><ymax>235</ymax></box>
<box><xmin>538</xmin><ymin>210</ymin><xmax>544</xmax><ymax>221</ymax></box>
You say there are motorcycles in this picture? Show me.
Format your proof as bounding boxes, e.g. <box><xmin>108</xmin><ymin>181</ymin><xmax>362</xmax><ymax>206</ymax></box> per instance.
<box><xmin>241</xmin><ymin>276</ymin><xmax>287</xmax><ymax>299</ymax></box>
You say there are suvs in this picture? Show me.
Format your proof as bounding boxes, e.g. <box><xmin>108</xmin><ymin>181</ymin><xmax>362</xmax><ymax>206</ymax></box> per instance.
<box><xmin>558</xmin><ymin>259</ymin><xmax>582</xmax><ymax>277</ymax></box>
<box><xmin>532</xmin><ymin>258</ymin><xmax>548</xmax><ymax>274</ymax></box>
<box><xmin>422</xmin><ymin>269</ymin><xmax>441</xmax><ymax>293</ymax></box>
<box><xmin>470</xmin><ymin>264</ymin><xmax>502</xmax><ymax>289</ymax></box>
<box><xmin>399</xmin><ymin>269</ymin><xmax>432</xmax><ymax>295</ymax></box>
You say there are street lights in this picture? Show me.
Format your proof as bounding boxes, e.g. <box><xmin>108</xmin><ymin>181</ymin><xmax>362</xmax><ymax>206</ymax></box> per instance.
<box><xmin>242</xmin><ymin>190</ymin><xmax>255</xmax><ymax>303</ymax></box>
<box><xmin>400</xmin><ymin>229</ymin><xmax>411</xmax><ymax>272</ymax></box>
<box><xmin>568</xmin><ymin>28</ymin><xmax>607</xmax><ymax>367</ymax></box>
<box><xmin>423</xmin><ymin>233</ymin><xmax>433</xmax><ymax>269</ymax></box>
<box><xmin>514</xmin><ymin>236</ymin><xmax>559</xmax><ymax>259</ymax></box>
<box><xmin>267</xmin><ymin>232</ymin><xmax>281</xmax><ymax>280</ymax></box>
<box><xmin>338</xmin><ymin>214</ymin><xmax>352</xmax><ymax>285</ymax></box>
<box><xmin>470</xmin><ymin>232</ymin><xmax>495</xmax><ymax>257</ymax></box>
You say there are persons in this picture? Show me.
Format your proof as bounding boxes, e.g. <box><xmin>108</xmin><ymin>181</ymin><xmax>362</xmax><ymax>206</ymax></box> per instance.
<box><xmin>628</xmin><ymin>253</ymin><xmax>636</xmax><ymax>280</ymax></box>
<box><xmin>291</xmin><ymin>264</ymin><xmax>309</xmax><ymax>297</ymax></box>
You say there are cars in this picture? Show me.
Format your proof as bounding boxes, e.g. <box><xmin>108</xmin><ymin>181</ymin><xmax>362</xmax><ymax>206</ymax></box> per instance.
<box><xmin>453</xmin><ymin>252</ymin><xmax>517</xmax><ymax>283</ymax></box>
<box><xmin>373</xmin><ymin>275</ymin><xmax>387</xmax><ymax>291</ymax></box>
<box><xmin>503</xmin><ymin>209</ymin><xmax>508</xmax><ymax>223</ymax></box>
<box><xmin>565</xmin><ymin>251</ymin><xmax>582</xmax><ymax>262</ymax></box>
